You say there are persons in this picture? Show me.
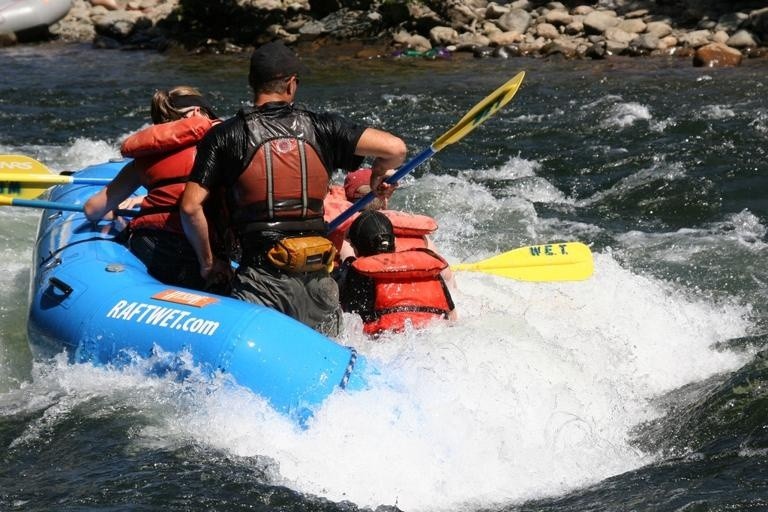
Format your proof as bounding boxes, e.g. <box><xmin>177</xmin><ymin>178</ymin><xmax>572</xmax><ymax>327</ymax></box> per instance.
<box><xmin>82</xmin><ymin>83</ymin><xmax>228</xmax><ymax>269</ymax></box>
<box><xmin>179</xmin><ymin>39</ymin><xmax>408</xmax><ymax>343</ymax></box>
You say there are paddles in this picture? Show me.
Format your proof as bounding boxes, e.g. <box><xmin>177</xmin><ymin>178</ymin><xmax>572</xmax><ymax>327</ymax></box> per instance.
<box><xmin>450</xmin><ymin>243</ymin><xmax>595</xmax><ymax>282</ymax></box>
<box><xmin>326</xmin><ymin>71</ymin><xmax>526</xmax><ymax>235</ymax></box>
<box><xmin>1</xmin><ymin>154</ymin><xmax>113</xmax><ymax>200</ymax></box>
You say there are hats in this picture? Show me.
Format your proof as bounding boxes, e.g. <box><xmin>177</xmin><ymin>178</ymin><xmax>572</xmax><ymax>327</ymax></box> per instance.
<box><xmin>343</xmin><ymin>168</ymin><xmax>386</xmax><ymax>201</ymax></box>
<box><xmin>165</xmin><ymin>95</ymin><xmax>221</xmax><ymax>122</ymax></box>
<box><xmin>248</xmin><ymin>41</ymin><xmax>298</xmax><ymax>80</ymax></box>
<box><xmin>344</xmin><ymin>209</ymin><xmax>393</xmax><ymax>245</ymax></box>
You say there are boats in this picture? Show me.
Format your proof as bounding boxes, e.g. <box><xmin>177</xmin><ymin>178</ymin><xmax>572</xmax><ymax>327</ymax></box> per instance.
<box><xmin>26</xmin><ymin>157</ymin><xmax>375</xmax><ymax>433</ymax></box>
<box><xmin>0</xmin><ymin>1</ymin><xmax>75</xmax><ymax>38</ymax></box>
